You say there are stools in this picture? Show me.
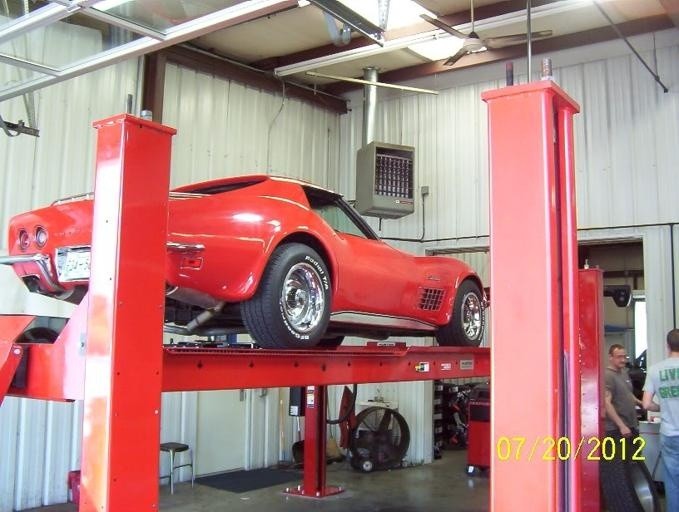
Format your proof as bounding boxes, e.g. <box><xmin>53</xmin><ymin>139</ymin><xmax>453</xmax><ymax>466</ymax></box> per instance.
<box><xmin>155</xmin><ymin>438</ymin><xmax>195</xmax><ymax>498</ymax></box>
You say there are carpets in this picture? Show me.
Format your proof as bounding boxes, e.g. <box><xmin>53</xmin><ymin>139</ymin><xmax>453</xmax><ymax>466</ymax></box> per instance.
<box><xmin>189</xmin><ymin>468</ymin><xmax>303</xmax><ymax>495</ymax></box>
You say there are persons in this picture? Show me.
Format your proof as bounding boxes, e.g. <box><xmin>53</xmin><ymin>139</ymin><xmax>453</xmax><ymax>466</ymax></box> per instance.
<box><xmin>605</xmin><ymin>343</ymin><xmax>643</xmax><ymax>438</ymax></box>
<box><xmin>644</xmin><ymin>328</ymin><xmax>679</xmax><ymax>512</ymax></box>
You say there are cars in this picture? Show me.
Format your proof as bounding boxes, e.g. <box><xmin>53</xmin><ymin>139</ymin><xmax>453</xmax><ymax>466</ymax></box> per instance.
<box><xmin>624</xmin><ymin>349</ymin><xmax>647</xmax><ymax>400</ymax></box>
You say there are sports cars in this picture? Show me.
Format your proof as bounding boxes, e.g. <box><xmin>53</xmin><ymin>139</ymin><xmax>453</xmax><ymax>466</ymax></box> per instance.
<box><xmin>0</xmin><ymin>174</ymin><xmax>487</xmax><ymax>349</ymax></box>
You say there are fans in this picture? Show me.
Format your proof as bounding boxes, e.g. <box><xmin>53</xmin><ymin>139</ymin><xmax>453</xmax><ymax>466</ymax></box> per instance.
<box><xmin>417</xmin><ymin>0</ymin><xmax>555</xmax><ymax>69</ymax></box>
